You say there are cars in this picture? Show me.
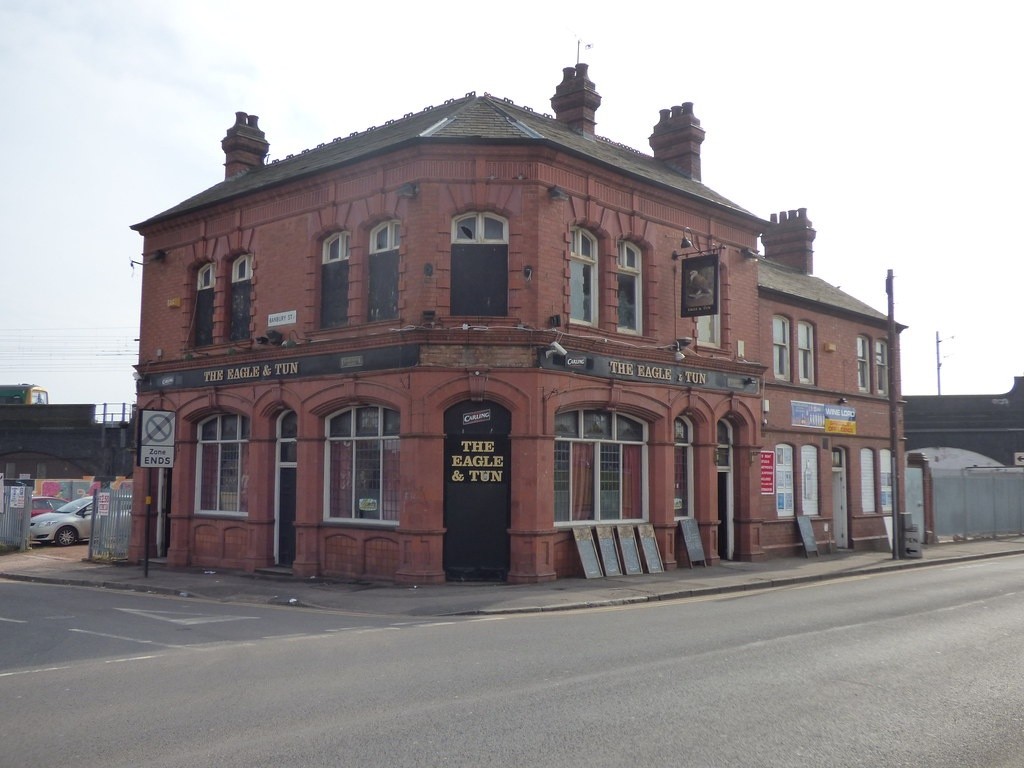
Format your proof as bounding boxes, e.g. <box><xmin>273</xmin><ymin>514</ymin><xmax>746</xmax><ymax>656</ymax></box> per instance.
<box><xmin>31</xmin><ymin>497</ymin><xmax>80</xmax><ymax>518</ymax></box>
<box><xmin>30</xmin><ymin>494</ymin><xmax>132</xmax><ymax>547</ymax></box>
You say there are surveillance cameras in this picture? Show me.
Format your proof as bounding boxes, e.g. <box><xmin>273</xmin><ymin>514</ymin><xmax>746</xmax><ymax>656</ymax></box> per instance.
<box><xmin>133</xmin><ymin>372</ymin><xmax>142</xmax><ymax>381</ymax></box>
<box><xmin>550</xmin><ymin>341</ymin><xmax>567</xmax><ymax>356</ymax></box>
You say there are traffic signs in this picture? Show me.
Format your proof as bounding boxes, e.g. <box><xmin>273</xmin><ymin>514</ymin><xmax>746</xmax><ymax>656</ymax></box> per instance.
<box><xmin>905</xmin><ymin>529</ymin><xmax>922</xmax><ymax>558</ymax></box>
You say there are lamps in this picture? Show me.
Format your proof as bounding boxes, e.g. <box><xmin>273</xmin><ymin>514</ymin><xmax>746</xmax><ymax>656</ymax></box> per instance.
<box><xmin>549</xmin><ymin>184</ymin><xmax>567</xmax><ymax>200</ymax></box>
<box><xmin>673</xmin><ymin>341</ymin><xmax>685</xmax><ymax>361</ymax></box>
<box><xmin>745</xmin><ymin>378</ymin><xmax>756</xmax><ymax>385</ymax></box>
<box><xmin>282</xmin><ymin>329</ymin><xmax>308</xmax><ymax>348</ymax></box>
<box><xmin>681</xmin><ymin>226</ymin><xmax>702</xmax><ymax>253</ymax></box>
<box><xmin>839</xmin><ymin>397</ymin><xmax>848</xmax><ymax>405</ymax></box>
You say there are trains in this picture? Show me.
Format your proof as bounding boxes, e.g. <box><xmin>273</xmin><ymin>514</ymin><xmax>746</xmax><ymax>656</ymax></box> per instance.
<box><xmin>0</xmin><ymin>384</ymin><xmax>49</xmax><ymax>404</ymax></box>
